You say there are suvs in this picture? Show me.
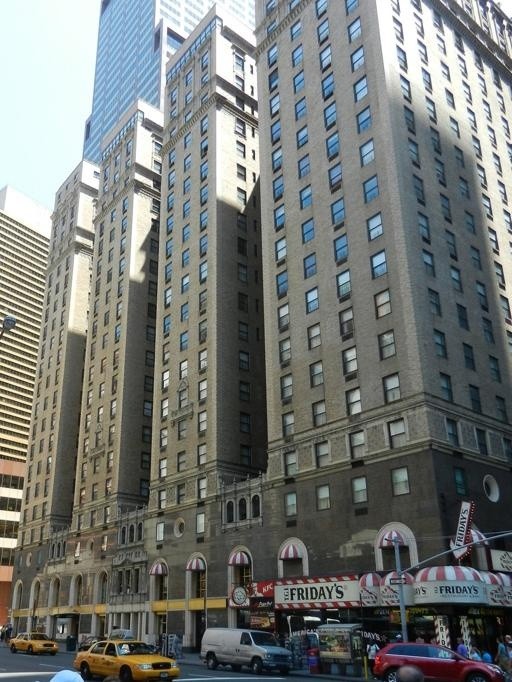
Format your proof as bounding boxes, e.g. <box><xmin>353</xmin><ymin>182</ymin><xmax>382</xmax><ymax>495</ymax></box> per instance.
<box><xmin>371</xmin><ymin>642</ymin><xmax>507</xmax><ymax>682</ymax></box>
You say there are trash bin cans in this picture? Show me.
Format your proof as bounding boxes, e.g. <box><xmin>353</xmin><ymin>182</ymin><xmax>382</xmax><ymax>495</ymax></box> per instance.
<box><xmin>66</xmin><ymin>636</ymin><xmax>77</xmax><ymax>651</ymax></box>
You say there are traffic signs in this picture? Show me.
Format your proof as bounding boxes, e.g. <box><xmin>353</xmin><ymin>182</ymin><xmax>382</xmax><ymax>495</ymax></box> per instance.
<box><xmin>390</xmin><ymin>577</ymin><xmax>406</xmax><ymax>584</ymax></box>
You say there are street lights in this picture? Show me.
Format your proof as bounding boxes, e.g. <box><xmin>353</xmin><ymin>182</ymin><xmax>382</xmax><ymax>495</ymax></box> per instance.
<box><xmin>0</xmin><ymin>316</ymin><xmax>17</xmax><ymax>339</ymax></box>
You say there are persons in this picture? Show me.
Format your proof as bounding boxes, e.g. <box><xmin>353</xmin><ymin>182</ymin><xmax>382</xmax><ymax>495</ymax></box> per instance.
<box><xmin>366</xmin><ymin>639</ymin><xmax>381</xmax><ymax>679</ymax></box>
<box><xmin>469</xmin><ymin>646</ymin><xmax>481</xmax><ymax>661</ymax></box>
<box><xmin>455</xmin><ymin>637</ymin><xmax>468</xmax><ymax>660</ymax></box>
<box><xmin>250</xmin><ymin>582</ymin><xmax>265</xmax><ymax>596</ymax></box>
<box><xmin>507</xmin><ymin>645</ymin><xmax>512</xmax><ymax>656</ymax></box>
<box><xmin>505</xmin><ymin>634</ymin><xmax>512</xmax><ymax>642</ymax></box>
<box><xmin>494</xmin><ymin>636</ymin><xmax>508</xmax><ymax>671</ymax></box>
<box><xmin>395</xmin><ymin>663</ymin><xmax>424</xmax><ymax>682</ymax></box>
<box><xmin>482</xmin><ymin>649</ymin><xmax>491</xmax><ymax>663</ymax></box>
<box><xmin>0</xmin><ymin>624</ymin><xmax>7</xmax><ymax>641</ymax></box>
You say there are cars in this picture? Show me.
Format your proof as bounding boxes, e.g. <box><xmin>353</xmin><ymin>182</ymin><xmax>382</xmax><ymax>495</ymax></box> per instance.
<box><xmin>70</xmin><ymin>638</ymin><xmax>182</xmax><ymax>682</ymax></box>
<box><xmin>7</xmin><ymin>632</ymin><xmax>58</xmax><ymax>657</ymax></box>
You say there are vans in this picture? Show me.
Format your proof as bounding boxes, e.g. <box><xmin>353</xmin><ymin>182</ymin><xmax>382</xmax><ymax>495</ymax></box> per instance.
<box><xmin>199</xmin><ymin>627</ymin><xmax>295</xmax><ymax>677</ymax></box>
